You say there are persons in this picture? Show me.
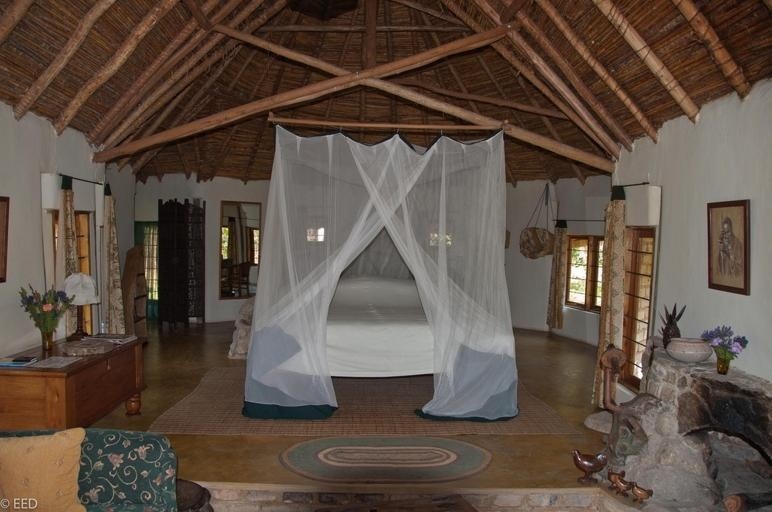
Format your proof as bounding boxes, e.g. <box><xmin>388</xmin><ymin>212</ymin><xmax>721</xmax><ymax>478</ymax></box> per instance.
<box><xmin>718</xmin><ymin>218</ymin><xmax>745</xmax><ymax>276</ymax></box>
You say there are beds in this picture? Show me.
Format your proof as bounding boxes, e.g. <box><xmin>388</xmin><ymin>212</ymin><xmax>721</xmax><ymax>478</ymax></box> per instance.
<box><xmin>249</xmin><ymin>277</ymin><xmax>515</xmax><ymax>379</ymax></box>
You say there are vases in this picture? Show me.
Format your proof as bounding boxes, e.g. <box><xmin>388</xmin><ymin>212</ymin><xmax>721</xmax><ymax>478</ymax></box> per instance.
<box><xmin>41</xmin><ymin>332</ymin><xmax>54</xmax><ymax>349</ymax></box>
<box><xmin>716</xmin><ymin>356</ymin><xmax>730</xmax><ymax>375</ymax></box>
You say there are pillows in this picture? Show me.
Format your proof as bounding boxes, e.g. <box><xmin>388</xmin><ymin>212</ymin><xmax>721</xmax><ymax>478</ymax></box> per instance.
<box><xmin>0</xmin><ymin>426</ymin><xmax>86</xmax><ymax>512</ymax></box>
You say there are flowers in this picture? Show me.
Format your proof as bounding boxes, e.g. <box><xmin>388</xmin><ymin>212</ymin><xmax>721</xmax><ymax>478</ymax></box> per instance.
<box><xmin>699</xmin><ymin>325</ymin><xmax>750</xmax><ymax>364</ymax></box>
<box><xmin>16</xmin><ymin>283</ymin><xmax>78</xmax><ymax>338</ymax></box>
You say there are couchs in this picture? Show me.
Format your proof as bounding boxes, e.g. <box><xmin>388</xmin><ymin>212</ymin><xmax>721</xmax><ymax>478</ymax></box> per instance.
<box><xmin>0</xmin><ymin>425</ymin><xmax>212</xmax><ymax>512</ymax></box>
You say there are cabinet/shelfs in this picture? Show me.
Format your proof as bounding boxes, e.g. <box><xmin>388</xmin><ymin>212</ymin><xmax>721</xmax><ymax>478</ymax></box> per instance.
<box><xmin>121</xmin><ymin>245</ymin><xmax>157</xmax><ymax>349</ymax></box>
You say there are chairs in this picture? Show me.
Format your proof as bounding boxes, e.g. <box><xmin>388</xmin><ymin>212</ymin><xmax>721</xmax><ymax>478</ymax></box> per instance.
<box><xmin>227</xmin><ymin>261</ymin><xmax>251</xmax><ymax>298</ymax></box>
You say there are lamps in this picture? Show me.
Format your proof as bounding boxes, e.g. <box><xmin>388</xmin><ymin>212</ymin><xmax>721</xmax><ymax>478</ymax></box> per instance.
<box><xmin>62</xmin><ymin>272</ymin><xmax>102</xmax><ymax>342</ymax></box>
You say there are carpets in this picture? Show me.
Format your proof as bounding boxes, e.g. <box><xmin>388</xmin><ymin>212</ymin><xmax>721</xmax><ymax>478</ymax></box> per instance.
<box><xmin>186</xmin><ymin>477</ymin><xmax>635</xmax><ymax>512</ymax></box>
<box><xmin>278</xmin><ymin>435</ymin><xmax>495</xmax><ymax>484</ymax></box>
<box><xmin>148</xmin><ymin>364</ymin><xmax>588</xmax><ymax>435</ymax></box>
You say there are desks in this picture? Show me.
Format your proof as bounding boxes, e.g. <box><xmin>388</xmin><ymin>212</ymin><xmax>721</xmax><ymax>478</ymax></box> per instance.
<box><xmin>0</xmin><ymin>331</ymin><xmax>148</xmax><ymax>432</ymax></box>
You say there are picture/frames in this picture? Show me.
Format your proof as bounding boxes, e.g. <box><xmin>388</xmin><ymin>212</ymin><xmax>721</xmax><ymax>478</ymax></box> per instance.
<box><xmin>704</xmin><ymin>199</ymin><xmax>751</xmax><ymax>298</ymax></box>
<box><xmin>0</xmin><ymin>195</ymin><xmax>10</xmax><ymax>283</ymax></box>
